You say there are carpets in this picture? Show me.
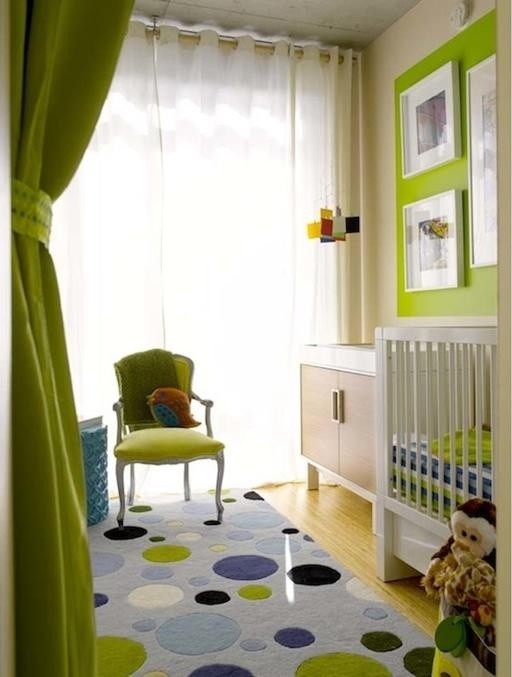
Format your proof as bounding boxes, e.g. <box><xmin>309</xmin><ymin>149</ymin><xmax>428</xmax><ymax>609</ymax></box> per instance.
<box><xmin>86</xmin><ymin>491</ymin><xmax>436</xmax><ymax>677</ymax></box>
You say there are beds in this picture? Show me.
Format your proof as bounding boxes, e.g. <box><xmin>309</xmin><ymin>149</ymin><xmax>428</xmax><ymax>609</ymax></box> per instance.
<box><xmin>375</xmin><ymin>324</ymin><xmax>500</xmax><ymax>583</ymax></box>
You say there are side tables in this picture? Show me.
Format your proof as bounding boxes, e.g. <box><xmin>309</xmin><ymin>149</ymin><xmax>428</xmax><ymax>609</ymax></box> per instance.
<box><xmin>77</xmin><ymin>413</ymin><xmax>110</xmax><ymax>526</ymax></box>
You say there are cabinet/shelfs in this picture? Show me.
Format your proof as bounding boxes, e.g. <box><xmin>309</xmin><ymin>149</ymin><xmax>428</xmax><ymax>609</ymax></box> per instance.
<box><xmin>298</xmin><ymin>341</ymin><xmax>478</xmax><ymax>536</ymax></box>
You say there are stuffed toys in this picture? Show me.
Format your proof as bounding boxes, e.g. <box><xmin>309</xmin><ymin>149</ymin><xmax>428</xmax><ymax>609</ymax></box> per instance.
<box><xmin>421</xmin><ymin>491</ymin><xmax>498</xmax><ymax>674</ymax></box>
<box><xmin>422</xmin><ymin>540</ymin><xmax>499</xmax><ymax>628</ymax></box>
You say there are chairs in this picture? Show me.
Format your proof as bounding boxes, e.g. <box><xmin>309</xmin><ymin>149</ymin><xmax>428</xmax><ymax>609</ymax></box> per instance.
<box><xmin>112</xmin><ymin>349</ymin><xmax>224</xmax><ymax>530</ymax></box>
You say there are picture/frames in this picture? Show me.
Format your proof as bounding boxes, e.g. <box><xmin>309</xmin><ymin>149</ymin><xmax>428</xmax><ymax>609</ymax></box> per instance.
<box><xmin>399</xmin><ymin>60</ymin><xmax>462</xmax><ymax>179</ymax></box>
<box><xmin>402</xmin><ymin>189</ymin><xmax>464</xmax><ymax>292</ymax></box>
<box><xmin>466</xmin><ymin>52</ymin><xmax>498</xmax><ymax>268</ymax></box>
